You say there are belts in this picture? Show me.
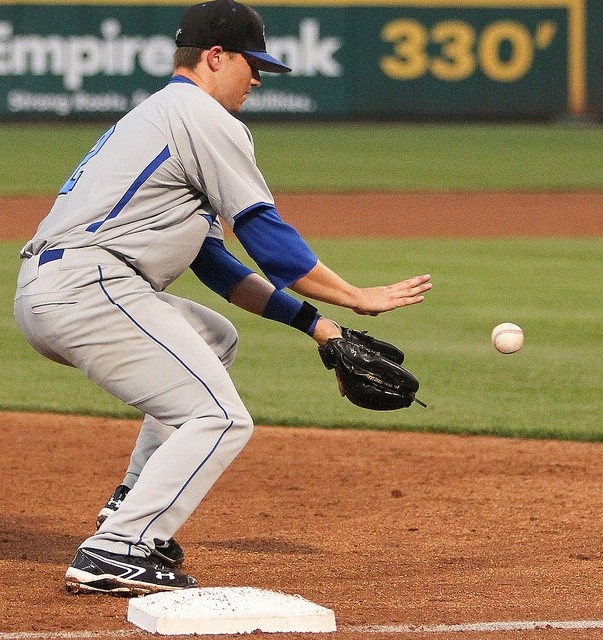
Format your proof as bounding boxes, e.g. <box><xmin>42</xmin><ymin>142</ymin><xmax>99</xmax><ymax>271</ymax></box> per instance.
<box><xmin>38</xmin><ymin>248</ymin><xmax>65</xmax><ymax>266</ymax></box>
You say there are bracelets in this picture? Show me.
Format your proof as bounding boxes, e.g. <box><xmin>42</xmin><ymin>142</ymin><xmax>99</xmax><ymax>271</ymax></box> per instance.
<box><xmin>262</xmin><ymin>286</ymin><xmax>322</xmax><ymax>336</ymax></box>
<box><xmin>291</xmin><ymin>301</ymin><xmax>318</xmax><ymax>333</ymax></box>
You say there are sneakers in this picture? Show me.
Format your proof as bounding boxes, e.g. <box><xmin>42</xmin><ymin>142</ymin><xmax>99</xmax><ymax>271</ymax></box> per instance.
<box><xmin>94</xmin><ymin>485</ymin><xmax>184</xmax><ymax>570</ymax></box>
<box><xmin>62</xmin><ymin>543</ymin><xmax>200</xmax><ymax>595</ymax></box>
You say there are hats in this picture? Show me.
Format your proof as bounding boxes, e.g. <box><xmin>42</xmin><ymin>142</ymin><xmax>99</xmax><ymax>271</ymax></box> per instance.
<box><xmin>175</xmin><ymin>0</ymin><xmax>292</xmax><ymax>75</ymax></box>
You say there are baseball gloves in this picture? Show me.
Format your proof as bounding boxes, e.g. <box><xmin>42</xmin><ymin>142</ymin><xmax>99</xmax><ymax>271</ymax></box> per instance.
<box><xmin>317</xmin><ymin>325</ymin><xmax>428</xmax><ymax>412</ymax></box>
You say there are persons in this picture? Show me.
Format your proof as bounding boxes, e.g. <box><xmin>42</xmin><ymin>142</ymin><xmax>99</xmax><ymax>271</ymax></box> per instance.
<box><xmin>12</xmin><ymin>0</ymin><xmax>433</xmax><ymax>597</ymax></box>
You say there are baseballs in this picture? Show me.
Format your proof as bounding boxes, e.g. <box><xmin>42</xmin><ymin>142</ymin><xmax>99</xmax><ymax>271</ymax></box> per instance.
<box><xmin>490</xmin><ymin>321</ymin><xmax>525</xmax><ymax>355</ymax></box>
